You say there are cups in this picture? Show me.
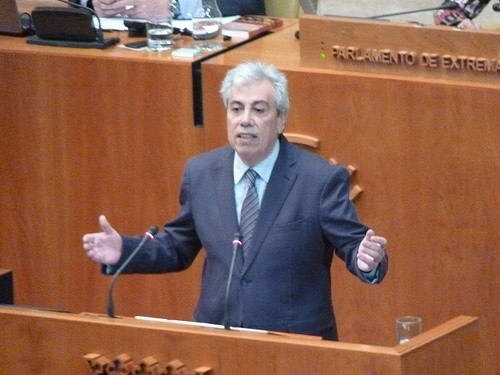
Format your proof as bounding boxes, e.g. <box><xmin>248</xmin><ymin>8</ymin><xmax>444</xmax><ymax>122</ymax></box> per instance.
<box><xmin>395</xmin><ymin>315</ymin><xmax>422</xmax><ymax>347</ymax></box>
<box><xmin>145</xmin><ymin>10</ymin><xmax>174</xmax><ymax>51</ymax></box>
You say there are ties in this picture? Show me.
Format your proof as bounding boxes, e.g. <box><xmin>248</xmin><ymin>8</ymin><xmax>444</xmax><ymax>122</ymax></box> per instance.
<box><xmin>241</xmin><ymin>169</ymin><xmax>260</xmax><ymax>261</ymax></box>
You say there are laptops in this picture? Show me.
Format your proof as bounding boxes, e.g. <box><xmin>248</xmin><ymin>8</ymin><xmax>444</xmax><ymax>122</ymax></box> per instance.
<box><xmin>0</xmin><ymin>0</ymin><xmax>35</xmax><ymax>35</ymax></box>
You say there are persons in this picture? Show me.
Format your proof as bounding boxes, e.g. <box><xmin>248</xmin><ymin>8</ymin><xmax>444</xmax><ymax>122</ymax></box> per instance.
<box><xmin>83</xmin><ymin>61</ymin><xmax>389</xmax><ymax>341</ymax></box>
<box><xmin>68</xmin><ymin>0</ymin><xmax>266</xmax><ymax>21</ymax></box>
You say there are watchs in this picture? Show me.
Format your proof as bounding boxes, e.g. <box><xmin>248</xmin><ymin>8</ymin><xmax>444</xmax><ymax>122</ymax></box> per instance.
<box><xmin>169</xmin><ymin>0</ymin><xmax>181</xmax><ymax>19</ymax></box>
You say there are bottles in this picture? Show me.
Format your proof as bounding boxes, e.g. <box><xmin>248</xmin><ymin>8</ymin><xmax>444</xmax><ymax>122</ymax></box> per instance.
<box><xmin>186</xmin><ymin>0</ymin><xmax>223</xmax><ymax>50</ymax></box>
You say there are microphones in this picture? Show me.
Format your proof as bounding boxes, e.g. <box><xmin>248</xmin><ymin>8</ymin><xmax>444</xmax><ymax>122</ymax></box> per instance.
<box><xmin>107</xmin><ymin>225</ymin><xmax>158</xmax><ymax>318</ymax></box>
<box><xmin>224</xmin><ymin>232</ymin><xmax>243</xmax><ymax>330</ymax></box>
